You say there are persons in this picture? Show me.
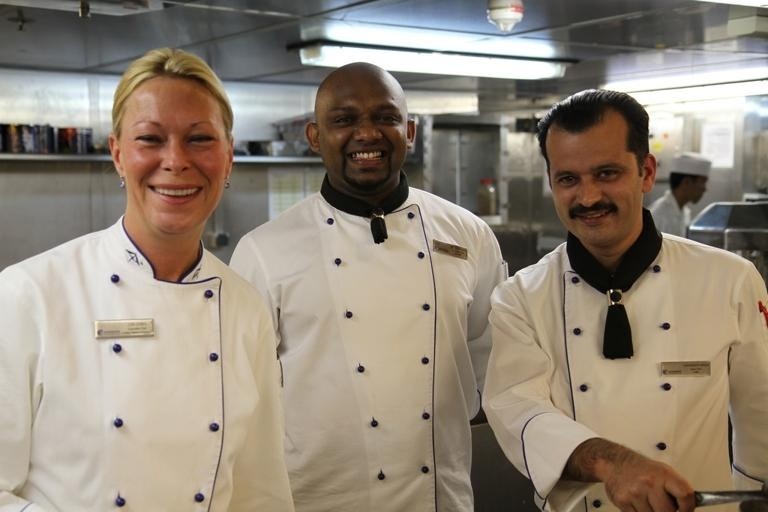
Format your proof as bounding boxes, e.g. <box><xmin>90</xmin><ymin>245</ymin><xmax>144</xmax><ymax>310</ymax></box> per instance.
<box><xmin>0</xmin><ymin>42</ymin><xmax>294</xmax><ymax>511</ymax></box>
<box><xmin>227</xmin><ymin>60</ymin><xmax>516</xmax><ymax>512</ymax></box>
<box><xmin>478</xmin><ymin>88</ymin><xmax>768</xmax><ymax>511</ymax></box>
<box><xmin>648</xmin><ymin>151</ymin><xmax>714</xmax><ymax>238</ymax></box>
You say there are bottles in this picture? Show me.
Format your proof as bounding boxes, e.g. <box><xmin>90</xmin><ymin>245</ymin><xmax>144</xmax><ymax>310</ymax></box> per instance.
<box><xmin>474</xmin><ymin>176</ymin><xmax>497</xmax><ymax>216</ymax></box>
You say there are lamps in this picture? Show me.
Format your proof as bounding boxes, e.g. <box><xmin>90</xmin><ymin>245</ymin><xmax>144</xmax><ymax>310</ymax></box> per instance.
<box><xmin>286</xmin><ymin>22</ymin><xmax>582</xmax><ymax>81</ymax></box>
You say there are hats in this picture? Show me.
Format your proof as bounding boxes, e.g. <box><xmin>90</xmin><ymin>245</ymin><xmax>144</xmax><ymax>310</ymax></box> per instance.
<box><xmin>670</xmin><ymin>153</ymin><xmax>712</xmax><ymax>178</ymax></box>
